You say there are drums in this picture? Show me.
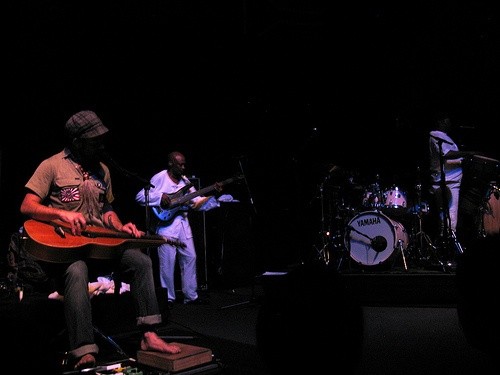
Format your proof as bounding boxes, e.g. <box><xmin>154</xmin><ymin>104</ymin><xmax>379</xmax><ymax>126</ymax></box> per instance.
<box><xmin>343</xmin><ymin>210</ymin><xmax>410</xmax><ymax>266</ymax></box>
<box><xmin>383</xmin><ymin>186</ymin><xmax>408</xmax><ymax>209</ymax></box>
<box><xmin>361</xmin><ymin>190</ymin><xmax>383</xmax><ymax>208</ymax></box>
<box><xmin>481</xmin><ymin>184</ymin><xmax>500</xmax><ymax>235</ymax></box>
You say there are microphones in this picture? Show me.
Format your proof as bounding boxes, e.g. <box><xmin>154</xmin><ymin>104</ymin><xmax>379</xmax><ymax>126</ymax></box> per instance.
<box><xmin>101</xmin><ymin>151</ymin><xmax>130</xmax><ymax>177</ymax></box>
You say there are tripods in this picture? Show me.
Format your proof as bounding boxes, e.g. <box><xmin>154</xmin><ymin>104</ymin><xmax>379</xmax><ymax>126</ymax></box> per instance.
<box><xmin>409</xmin><ymin>141</ymin><xmax>465</xmax><ymax>273</ymax></box>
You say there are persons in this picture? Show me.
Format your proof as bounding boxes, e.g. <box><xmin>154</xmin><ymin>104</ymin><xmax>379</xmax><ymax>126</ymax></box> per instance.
<box><xmin>135</xmin><ymin>151</ymin><xmax>223</xmax><ymax>308</ymax></box>
<box><xmin>428</xmin><ymin>117</ymin><xmax>465</xmax><ymax>254</ymax></box>
<box><xmin>19</xmin><ymin>109</ymin><xmax>181</xmax><ymax>369</ymax></box>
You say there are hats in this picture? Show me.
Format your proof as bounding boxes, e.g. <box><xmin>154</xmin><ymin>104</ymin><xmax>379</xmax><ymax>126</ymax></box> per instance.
<box><xmin>65</xmin><ymin>110</ymin><xmax>109</xmax><ymax>139</ymax></box>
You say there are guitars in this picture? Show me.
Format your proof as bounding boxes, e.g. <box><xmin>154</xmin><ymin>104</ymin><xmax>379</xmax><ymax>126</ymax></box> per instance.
<box><xmin>152</xmin><ymin>173</ymin><xmax>246</xmax><ymax>222</ymax></box>
<box><xmin>20</xmin><ymin>218</ymin><xmax>186</xmax><ymax>264</ymax></box>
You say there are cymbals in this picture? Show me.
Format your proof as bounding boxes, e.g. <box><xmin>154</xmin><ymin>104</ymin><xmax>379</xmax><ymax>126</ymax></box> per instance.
<box><xmin>433</xmin><ymin>181</ymin><xmax>460</xmax><ymax>185</ymax></box>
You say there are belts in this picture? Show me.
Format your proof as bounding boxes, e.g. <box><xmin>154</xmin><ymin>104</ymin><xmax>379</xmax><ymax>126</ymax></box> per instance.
<box><xmin>434</xmin><ymin>181</ymin><xmax>461</xmax><ymax>185</ymax></box>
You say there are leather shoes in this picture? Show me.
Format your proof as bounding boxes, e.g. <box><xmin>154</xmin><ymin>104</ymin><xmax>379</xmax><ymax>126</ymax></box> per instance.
<box><xmin>186</xmin><ymin>297</ymin><xmax>210</xmax><ymax>305</ymax></box>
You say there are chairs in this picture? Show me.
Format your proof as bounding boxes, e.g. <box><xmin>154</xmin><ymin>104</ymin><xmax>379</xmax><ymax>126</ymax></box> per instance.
<box><xmin>49</xmin><ymin>261</ymin><xmax>131</xmax><ymax>368</ymax></box>
